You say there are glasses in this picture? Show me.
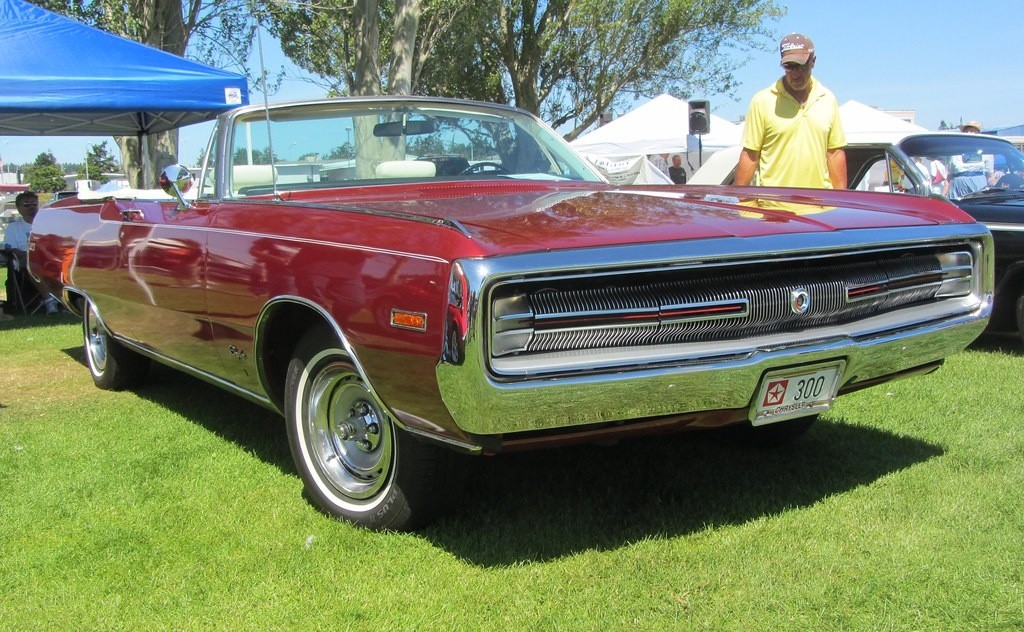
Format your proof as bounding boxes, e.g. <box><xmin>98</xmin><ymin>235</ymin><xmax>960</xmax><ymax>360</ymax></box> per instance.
<box><xmin>781</xmin><ymin>56</ymin><xmax>813</xmax><ymax>72</ymax></box>
<box><xmin>18</xmin><ymin>201</ymin><xmax>39</xmax><ymax>207</ymax></box>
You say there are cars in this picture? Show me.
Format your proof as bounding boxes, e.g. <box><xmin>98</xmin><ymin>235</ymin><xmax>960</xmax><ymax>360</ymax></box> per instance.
<box><xmin>687</xmin><ymin>129</ymin><xmax>1023</xmax><ymax>345</ymax></box>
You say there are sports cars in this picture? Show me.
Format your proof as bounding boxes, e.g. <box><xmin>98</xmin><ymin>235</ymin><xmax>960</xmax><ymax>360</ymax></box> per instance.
<box><xmin>28</xmin><ymin>92</ymin><xmax>995</xmax><ymax>534</ymax></box>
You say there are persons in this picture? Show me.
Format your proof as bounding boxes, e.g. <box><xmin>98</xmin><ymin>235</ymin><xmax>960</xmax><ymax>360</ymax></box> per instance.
<box><xmin>170</xmin><ymin>236</ymin><xmax>210</xmax><ymax>293</ymax></box>
<box><xmin>733</xmin><ymin>32</ymin><xmax>851</xmax><ymax>191</ymax></box>
<box><xmin>905</xmin><ymin>157</ymin><xmax>931</xmax><ymax>195</ymax></box>
<box><xmin>649</xmin><ymin>153</ymin><xmax>670</xmax><ymax>178</ymax></box>
<box><xmin>248</xmin><ymin>235</ymin><xmax>311</xmax><ymax>303</ymax></box>
<box><xmin>926</xmin><ymin>153</ymin><xmax>948</xmax><ymax>194</ymax></box>
<box><xmin>2</xmin><ymin>187</ymin><xmax>67</xmax><ymax>315</ymax></box>
<box><xmin>287</xmin><ymin>240</ymin><xmax>413</xmax><ymax>345</ymax></box>
<box><xmin>940</xmin><ymin>121</ymin><xmax>998</xmax><ymax>196</ymax></box>
<box><xmin>882</xmin><ymin>155</ymin><xmax>905</xmax><ymax>191</ymax></box>
<box><xmin>988</xmin><ymin>164</ymin><xmax>1024</xmax><ymax>190</ymax></box>
<box><xmin>728</xmin><ymin>190</ymin><xmax>836</xmax><ymax>226</ymax></box>
<box><xmin>669</xmin><ymin>154</ymin><xmax>688</xmax><ymax>185</ymax></box>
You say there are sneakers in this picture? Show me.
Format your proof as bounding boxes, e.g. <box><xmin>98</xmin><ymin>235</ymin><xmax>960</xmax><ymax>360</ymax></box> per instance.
<box><xmin>43</xmin><ymin>299</ymin><xmax>59</xmax><ymax>315</ymax></box>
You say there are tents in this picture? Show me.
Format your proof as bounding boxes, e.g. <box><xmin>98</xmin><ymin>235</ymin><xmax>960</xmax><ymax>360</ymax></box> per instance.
<box><xmin>0</xmin><ymin>0</ymin><xmax>257</xmax><ymax>211</ymax></box>
<box><xmin>712</xmin><ymin>95</ymin><xmax>935</xmax><ymax>193</ymax></box>
<box><xmin>563</xmin><ymin>93</ymin><xmax>744</xmax><ymax>187</ymax></box>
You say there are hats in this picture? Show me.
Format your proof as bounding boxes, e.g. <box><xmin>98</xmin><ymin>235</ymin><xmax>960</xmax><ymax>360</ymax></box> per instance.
<box><xmin>780</xmin><ymin>32</ymin><xmax>815</xmax><ymax>66</ymax></box>
